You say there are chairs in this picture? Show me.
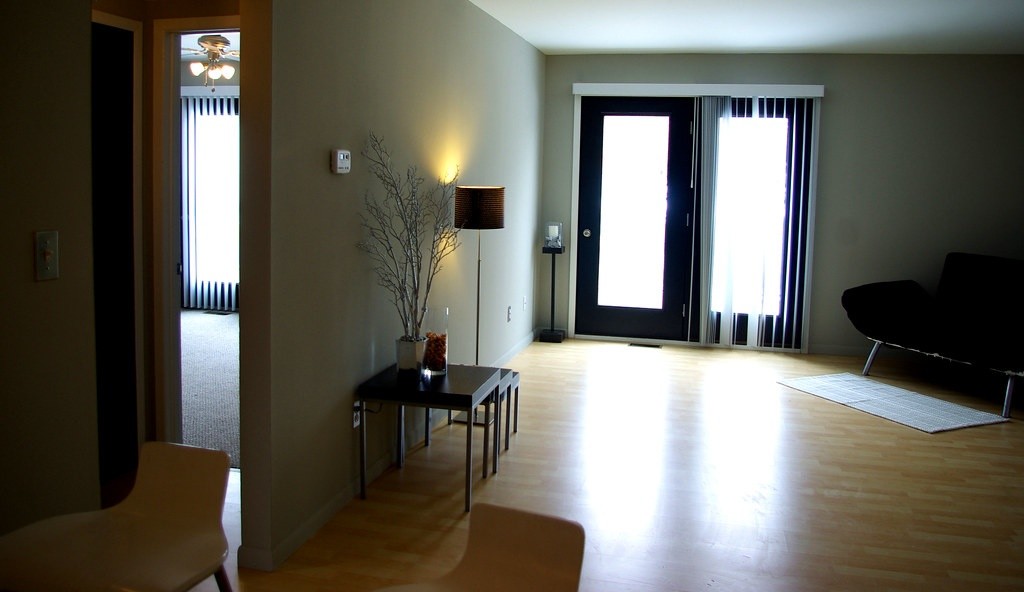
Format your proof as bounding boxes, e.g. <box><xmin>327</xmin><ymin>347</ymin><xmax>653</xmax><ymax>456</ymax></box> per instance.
<box><xmin>0</xmin><ymin>441</ymin><xmax>232</xmax><ymax>592</ymax></box>
<box><xmin>372</xmin><ymin>501</ymin><xmax>586</xmax><ymax>592</ymax></box>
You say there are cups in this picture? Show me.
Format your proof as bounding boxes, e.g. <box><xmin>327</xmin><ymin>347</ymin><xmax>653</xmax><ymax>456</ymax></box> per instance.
<box><xmin>423</xmin><ymin>306</ymin><xmax>450</xmax><ymax>375</ymax></box>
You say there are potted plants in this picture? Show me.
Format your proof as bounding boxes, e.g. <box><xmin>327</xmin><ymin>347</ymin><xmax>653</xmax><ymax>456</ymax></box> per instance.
<box><xmin>352</xmin><ymin>126</ymin><xmax>469</xmax><ymax>379</ymax></box>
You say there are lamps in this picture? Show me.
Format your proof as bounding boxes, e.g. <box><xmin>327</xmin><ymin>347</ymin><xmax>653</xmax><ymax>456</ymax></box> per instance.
<box><xmin>452</xmin><ymin>185</ymin><xmax>505</xmax><ymax>427</ymax></box>
<box><xmin>189</xmin><ymin>52</ymin><xmax>236</xmax><ymax>80</ymax></box>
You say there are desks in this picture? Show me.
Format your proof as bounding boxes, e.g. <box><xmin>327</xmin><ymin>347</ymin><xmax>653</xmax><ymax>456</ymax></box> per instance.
<box><xmin>354</xmin><ymin>362</ymin><xmax>520</xmax><ymax>513</ymax></box>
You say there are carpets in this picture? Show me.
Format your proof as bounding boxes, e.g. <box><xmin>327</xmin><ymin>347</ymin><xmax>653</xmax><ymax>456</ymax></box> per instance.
<box><xmin>775</xmin><ymin>371</ymin><xmax>1012</xmax><ymax>435</ymax></box>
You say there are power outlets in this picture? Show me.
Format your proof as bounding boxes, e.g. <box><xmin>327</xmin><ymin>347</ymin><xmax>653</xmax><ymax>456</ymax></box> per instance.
<box><xmin>523</xmin><ymin>296</ymin><xmax>527</xmax><ymax>310</ymax></box>
<box><xmin>508</xmin><ymin>306</ymin><xmax>512</xmax><ymax>322</ymax></box>
<box><xmin>353</xmin><ymin>401</ymin><xmax>360</xmax><ymax>428</ymax></box>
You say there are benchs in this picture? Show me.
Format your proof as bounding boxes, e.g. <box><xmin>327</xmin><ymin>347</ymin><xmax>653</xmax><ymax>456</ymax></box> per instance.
<box><xmin>841</xmin><ymin>251</ymin><xmax>1024</xmax><ymax>418</ymax></box>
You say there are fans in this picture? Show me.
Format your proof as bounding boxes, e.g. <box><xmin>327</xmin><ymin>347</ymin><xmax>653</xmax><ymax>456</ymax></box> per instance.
<box><xmin>181</xmin><ymin>35</ymin><xmax>240</xmax><ymax>58</ymax></box>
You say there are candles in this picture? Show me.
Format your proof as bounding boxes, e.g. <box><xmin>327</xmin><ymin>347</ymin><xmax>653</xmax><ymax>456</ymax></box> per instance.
<box><xmin>544</xmin><ymin>220</ymin><xmax>563</xmax><ymax>248</ymax></box>
